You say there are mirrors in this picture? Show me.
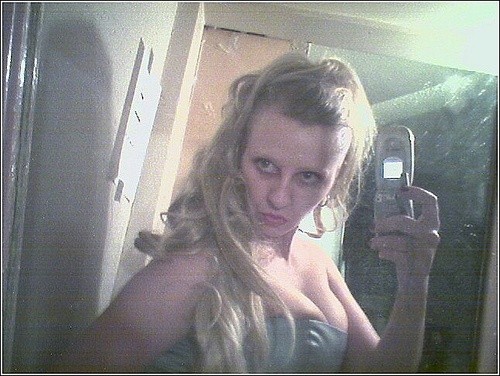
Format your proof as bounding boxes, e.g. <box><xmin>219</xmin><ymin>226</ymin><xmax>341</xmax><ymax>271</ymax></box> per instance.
<box><xmin>138</xmin><ymin>24</ymin><xmax>496</xmax><ymax>373</ymax></box>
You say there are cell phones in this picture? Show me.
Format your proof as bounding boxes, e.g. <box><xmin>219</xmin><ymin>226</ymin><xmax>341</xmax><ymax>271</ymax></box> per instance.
<box><xmin>373</xmin><ymin>125</ymin><xmax>415</xmax><ymax>225</ymax></box>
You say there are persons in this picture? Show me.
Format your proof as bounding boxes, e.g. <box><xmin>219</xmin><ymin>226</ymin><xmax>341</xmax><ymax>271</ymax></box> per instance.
<box><xmin>47</xmin><ymin>50</ymin><xmax>443</xmax><ymax>373</ymax></box>
